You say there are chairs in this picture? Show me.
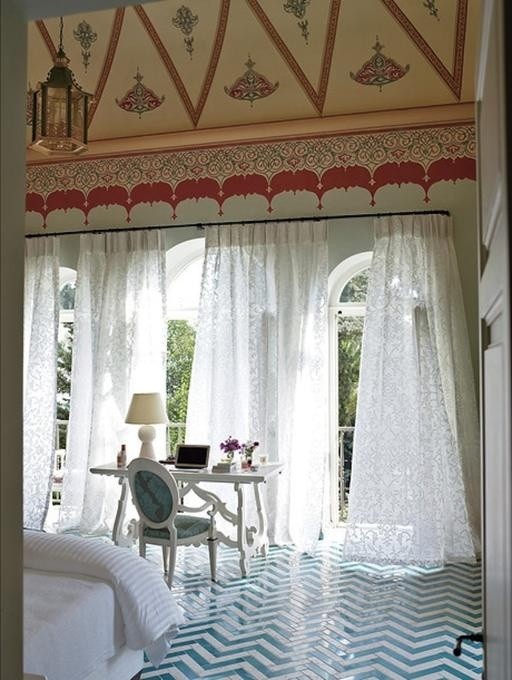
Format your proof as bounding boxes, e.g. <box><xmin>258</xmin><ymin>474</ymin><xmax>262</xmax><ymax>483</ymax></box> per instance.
<box><xmin>126</xmin><ymin>457</ymin><xmax>220</xmax><ymax>590</ymax></box>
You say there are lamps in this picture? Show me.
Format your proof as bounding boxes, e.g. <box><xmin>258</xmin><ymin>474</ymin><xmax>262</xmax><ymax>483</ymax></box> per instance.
<box><xmin>125</xmin><ymin>393</ymin><xmax>169</xmax><ymax>462</ymax></box>
<box><xmin>26</xmin><ymin>16</ymin><xmax>93</xmax><ymax>158</ymax></box>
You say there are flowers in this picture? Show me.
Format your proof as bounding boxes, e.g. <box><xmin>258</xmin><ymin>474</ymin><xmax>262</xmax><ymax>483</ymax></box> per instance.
<box><xmin>239</xmin><ymin>441</ymin><xmax>259</xmax><ymax>463</ymax></box>
<box><xmin>220</xmin><ymin>436</ymin><xmax>241</xmax><ymax>458</ymax></box>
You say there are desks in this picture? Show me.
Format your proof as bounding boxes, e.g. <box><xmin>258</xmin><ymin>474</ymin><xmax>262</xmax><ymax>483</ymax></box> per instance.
<box><xmin>90</xmin><ymin>462</ymin><xmax>286</xmax><ymax>578</ymax></box>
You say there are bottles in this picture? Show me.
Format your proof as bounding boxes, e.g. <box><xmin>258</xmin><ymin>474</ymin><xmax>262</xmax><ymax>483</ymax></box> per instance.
<box><xmin>117</xmin><ymin>444</ymin><xmax>127</xmax><ymax>468</ymax></box>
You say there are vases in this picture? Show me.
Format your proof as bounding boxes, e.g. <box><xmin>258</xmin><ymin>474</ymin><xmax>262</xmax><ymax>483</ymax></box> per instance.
<box><xmin>242</xmin><ymin>461</ymin><xmax>252</xmax><ymax>469</ymax></box>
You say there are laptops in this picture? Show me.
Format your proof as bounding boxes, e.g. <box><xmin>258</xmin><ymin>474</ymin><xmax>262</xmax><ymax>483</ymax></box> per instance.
<box><xmin>170</xmin><ymin>444</ymin><xmax>210</xmax><ymax>473</ymax></box>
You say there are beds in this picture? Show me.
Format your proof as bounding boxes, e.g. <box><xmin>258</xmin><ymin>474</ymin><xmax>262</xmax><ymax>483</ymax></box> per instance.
<box><xmin>23</xmin><ymin>529</ymin><xmax>186</xmax><ymax>680</ymax></box>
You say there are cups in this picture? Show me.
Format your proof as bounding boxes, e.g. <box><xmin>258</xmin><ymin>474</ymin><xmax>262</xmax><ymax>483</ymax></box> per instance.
<box><xmin>260</xmin><ymin>455</ymin><xmax>268</xmax><ymax>465</ymax></box>
<box><xmin>250</xmin><ymin>466</ymin><xmax>258</xmax><ymax>472</ymax></box>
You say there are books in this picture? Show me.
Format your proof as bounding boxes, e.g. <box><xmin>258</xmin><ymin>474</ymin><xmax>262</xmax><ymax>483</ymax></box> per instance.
<box><xmin>212</xmin><ymin>465</ymin><xmax>240</xmax><ymax>473</ymax></box>
<box><xmin>217</xmin><ymin>462</ymin><xmax>237</xmax><ymax>468</ymax></box>
